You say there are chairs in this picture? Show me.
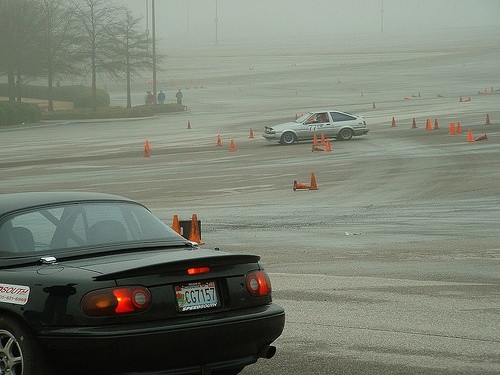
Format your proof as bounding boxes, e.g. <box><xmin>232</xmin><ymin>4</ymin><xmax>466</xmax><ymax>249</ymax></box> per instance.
<box><xmin>11</xmin><ymin>226</ymin><xmax>35</xmax><ymax>251</ymax></box>
<box><xmin>87</xmin><ymin>219</ymin><xmax>129</xmax><ymax>245</ymax></box>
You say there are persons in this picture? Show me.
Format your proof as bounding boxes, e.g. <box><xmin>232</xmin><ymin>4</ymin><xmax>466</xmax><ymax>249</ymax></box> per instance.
<box><xmin>176</xmin><ymin>89</ymin><xmax>183</xmax><ymax>105</ymax></box>
<box><xmin>147</xmin><ymin>91</ymin><xmax>154</xmax><ymax>104</ymax></box>
<box><xmin>157</xmin><ymin>90</ymin><xmax>165</xmax><ymax>104</ymax></box>
<box><xmin>145</xmin><ymin>91</ymin><xmax>150</xmax><ymax>105</ymax></box>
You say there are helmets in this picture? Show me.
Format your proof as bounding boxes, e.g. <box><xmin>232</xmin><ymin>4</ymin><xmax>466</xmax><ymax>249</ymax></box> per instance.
<box><xmin>316</xmin><ymin>113</ymin><xmax>327</xmax><ymax>122</ymax></box>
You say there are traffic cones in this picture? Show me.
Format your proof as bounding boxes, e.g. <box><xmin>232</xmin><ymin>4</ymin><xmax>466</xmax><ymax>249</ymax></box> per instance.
<box><xmin>307</xmin><ymin>172</ymin><xmax>320</xmax><ymax>190</ymax></box>
<box><xmin>464</xmin><ymin>129</ymin><xmax>475</xmax><ymax>142</ymax></box>
<box><xmin>456</xmin><ymin>122</ymin><xmax>463</xmax><ymax>133</ymax></box>
<box><xmin>186</xmin><ymin>212</ymin><xmax>203</xmax><ymax>244</ymax></box>
<box><xmin>311</xmin><ymin>144</ymin><xmax>324</xmax><ymax>152</ymax></box>
<box><xmin>292</xmin><ymin>180</ymin><xmax>310</xmax><ymax>191</ymax></box>
<box><xmin>483</xmin><ymin>114</ymin><xmax>492</xmax><ymax>125</ymax></box>
<box><xmin>447</xmin><ymin>122</ymin><xmax>456</xmax><ymax>135</ymax></box>
<box><xmin>170</xmin><ymin>214</ymin><xmax>182</xmax><ymax>235</ymax></box>
<box><xmin>228</xmin><ymin>140</ymin><xmax>236</xmax><ymax>152</ymax></box>
<box><xmin>371</xmin><ymin>84</ymin><xmax>500</xmax><ymax>109</ymax></box>
<box><xmin>424</xmin><ymin>118</ymin><xmax>433</xmax><ymax>130</ymax></box>
<box><xmin>248</xmin><ymin>128</ymin><xmax>255</xmax><ymax>139</ymax></box>
<box><xmin>325</xmin><ymin>138</ymin><xmax>333</xmax><ymax>151</ymax></box>
<box><xmin>186</xmin><ymin>120</ymin><xmax>192</xmax><ymax>129</ymax></box>
<box><xmin>312</xmin><ymin>132</ymin><xmax>319</xmax><ymax>145</ymax></box>
<box><xmin>411</xmin><ymin>117</ymin><xmax>418</xmax><ymax>128</ymax></box>
<box><xmin>391</xmin><ymin>117</ymin><xmax>397</xmax><ymax>127</ymax></box>
<box><xmin>320</xmin><ymin>132</ymin><xmax>326</xmax><ymax>145</ymax></box>
<box><xmin>215</xmin><ymin>134</ymin><xmax>224</xmax><ymax>146</ymax></box>
<box><xmin>474</xmin><ymin>133</ymin><xmax>488</xmax><ymax>141</ymax></box>
<box><xmin>143</xmin><ymin>140</ymin><xmax>151</xmax><ymax>157</ymax></box>
<box><xmin>434</xmin><ymin>118</ymin><xmax>440</xmax><ymax>129</ymax></box>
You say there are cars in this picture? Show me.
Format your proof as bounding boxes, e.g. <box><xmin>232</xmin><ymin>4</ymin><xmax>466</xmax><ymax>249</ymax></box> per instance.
<box><xmin>263</xmin><ymin>110</ymin><xmax>370</xmax><ymax>146</ymax></box>
<box><xmin>0</xmin><ymin>190</ymin><xmax>287</xmax><ymax>375</ymax></box>
